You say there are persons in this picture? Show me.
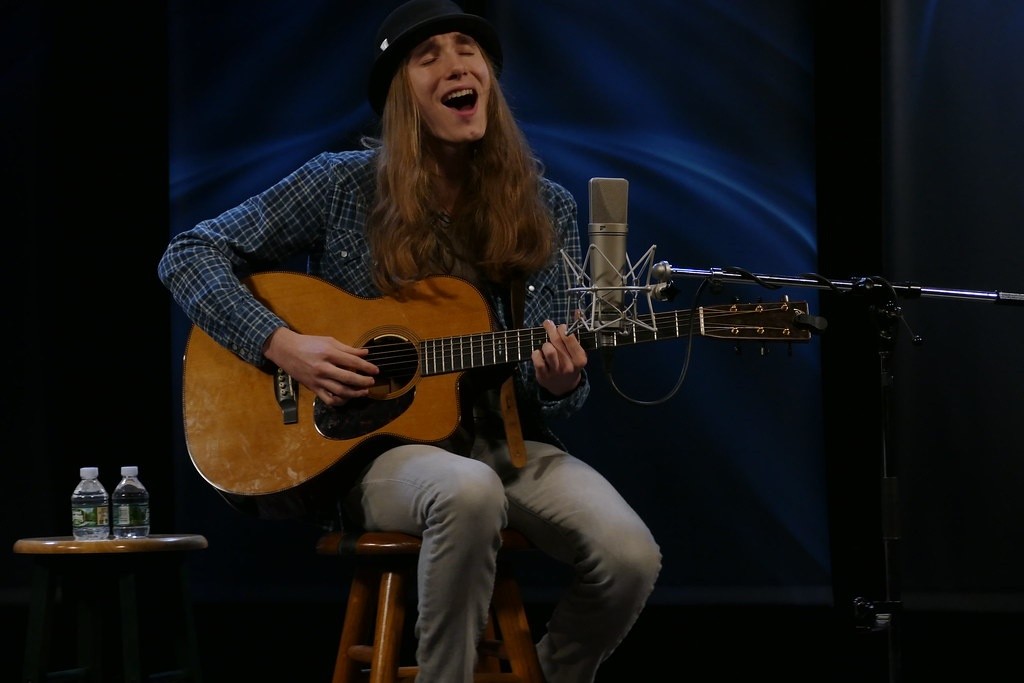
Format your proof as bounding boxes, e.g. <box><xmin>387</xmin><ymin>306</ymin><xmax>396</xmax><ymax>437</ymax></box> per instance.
<box><xmin>158</xmin><ymin>0</ymin><xmax>663</xmax><ymax>683</ymax></box>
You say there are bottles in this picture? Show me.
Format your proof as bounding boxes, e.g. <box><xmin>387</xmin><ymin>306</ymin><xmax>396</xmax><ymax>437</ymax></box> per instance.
<box><xmin>111</xmin><ymin>466</ymin><xmax>150</xmax><ymax>539</ymax></box>
<box><xmin>72</xmin><ymin>468</ymin><xmax>109</xmax><ymax>541</ymax></box>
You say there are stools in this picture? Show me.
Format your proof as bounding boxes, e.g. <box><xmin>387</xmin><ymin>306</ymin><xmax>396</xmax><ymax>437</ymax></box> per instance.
<box><xmin>332</xmin><ymin>531</ymin><xmax>542</xmax><ymax>683</ymax></box>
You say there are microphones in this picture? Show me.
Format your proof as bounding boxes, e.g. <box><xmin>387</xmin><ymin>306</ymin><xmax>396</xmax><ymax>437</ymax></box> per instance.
<box><xmin>588</xmin><ymin>178</ymin><xmax>629</xmax><ymax>368</ymax></box>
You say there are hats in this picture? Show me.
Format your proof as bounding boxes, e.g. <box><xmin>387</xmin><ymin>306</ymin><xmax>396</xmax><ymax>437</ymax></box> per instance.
<box><xmin>367</xmin><ymin>0</ymin><xmax>504</xmax><ymax>118</ymax></box>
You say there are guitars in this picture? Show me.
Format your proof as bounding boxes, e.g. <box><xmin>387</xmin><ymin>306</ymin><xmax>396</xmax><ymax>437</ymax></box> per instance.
<box><xmin>177</xmin><ymin>268</ymin><xmax>828</xmax><ymax>514</ymax></box>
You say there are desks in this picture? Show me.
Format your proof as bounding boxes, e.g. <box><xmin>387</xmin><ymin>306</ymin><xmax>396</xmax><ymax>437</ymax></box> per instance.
<box><xmin>12</xmin><ymin>532</ymin><xmax>207</xmax><ymax>683</ymax></box>
